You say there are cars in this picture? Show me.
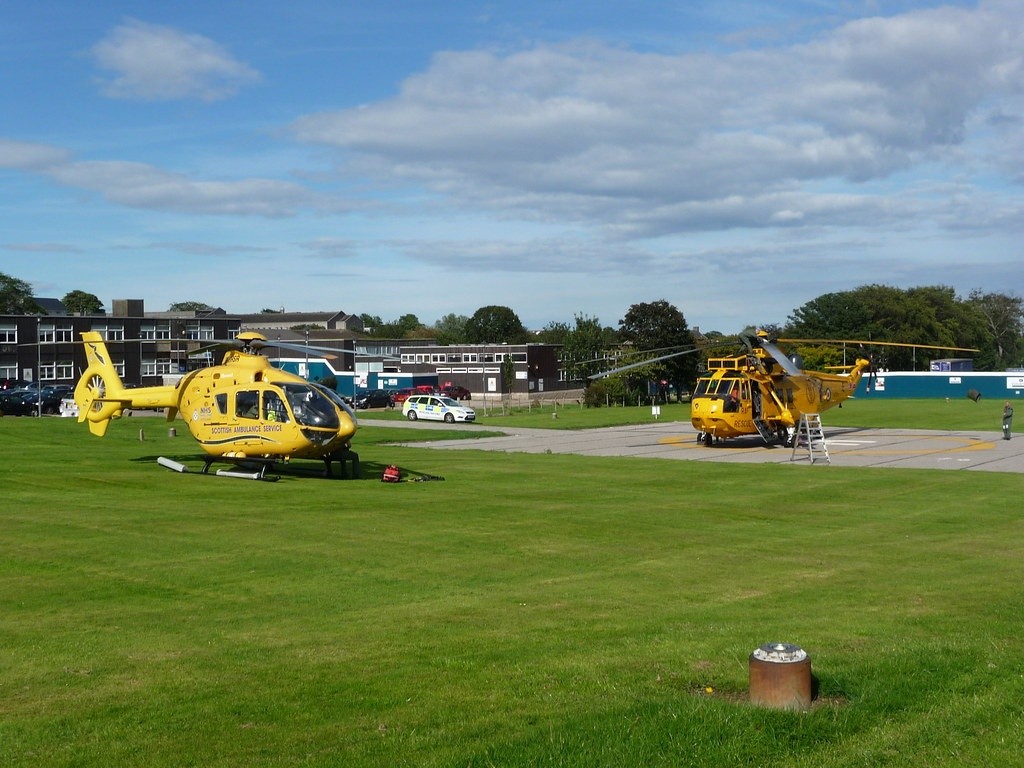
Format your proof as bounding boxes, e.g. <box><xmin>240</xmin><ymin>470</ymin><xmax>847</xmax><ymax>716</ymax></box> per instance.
<box><xmin>392</xmin><ymin>386</ymin><xmax>473</xmax><ymax>403</ymax></box>
<box><xmin>0</xmin><ymin>381</ymin><xmax>75</xmax><ymax>416</ymax></box>
<box><xmin>350</xmin><ymin>389</ymin><xmax>392</xmax><ymax>409</ymax></box>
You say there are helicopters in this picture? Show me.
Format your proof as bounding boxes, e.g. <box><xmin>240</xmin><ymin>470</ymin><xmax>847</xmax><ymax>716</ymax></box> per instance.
<box><xmin>74</xmin><ymin>329</ymin><xmax>373</xmax><ymax>480</ymax></box>
<box><xmin>573</xmin><ymin>336</ymin><xmax>981</xmax><ymax>448</ymax></box>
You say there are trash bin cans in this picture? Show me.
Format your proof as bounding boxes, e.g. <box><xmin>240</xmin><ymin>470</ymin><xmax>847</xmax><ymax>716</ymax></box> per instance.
<box><xmin>390</xmin><ymin>395</ymin><xmax>395</xmax><ymax>407</ymax></box>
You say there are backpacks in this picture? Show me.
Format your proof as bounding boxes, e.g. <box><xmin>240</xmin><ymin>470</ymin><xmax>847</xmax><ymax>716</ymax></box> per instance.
<box><xmin>382</xmin><ymin>464</ymin><xmax>401</xmax><ymax>482</ymax></box>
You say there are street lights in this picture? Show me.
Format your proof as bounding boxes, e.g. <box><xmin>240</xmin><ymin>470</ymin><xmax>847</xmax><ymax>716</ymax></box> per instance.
<box><xmin>277</xmin><ymin>337</ymin><xmax>282</xmax><ymax>371</ymax></box>
<box><xmin>351</xmin><ymin>338</ymin><xmax>358</xmax><ymax>411</ymax></box>
<box><xmin>35</xmin><ymin>315</ymin><xmax>44</xmax><ymax>418</ymax></box>
<box><xmin>303</xmin><ymin>329</ymin><xmax>310</xmax><ymax>382</ymax></box>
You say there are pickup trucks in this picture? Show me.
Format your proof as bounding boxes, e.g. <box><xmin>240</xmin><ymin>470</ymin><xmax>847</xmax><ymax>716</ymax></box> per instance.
<box><xmin>403</xmin><ymin>395</ymin><xmax>476</xmax><ymax>424</ymax></box>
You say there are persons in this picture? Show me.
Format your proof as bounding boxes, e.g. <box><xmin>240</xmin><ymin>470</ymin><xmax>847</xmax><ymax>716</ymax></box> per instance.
<box><xmin>1002</xmin><ymin>401</ymin><xmax>1014</xmax><ymax>440</ymax></box>
<box><xmin>731</xmin><ymin>385</ymin><xmax>740</xmax><ymax>412</ymax></box>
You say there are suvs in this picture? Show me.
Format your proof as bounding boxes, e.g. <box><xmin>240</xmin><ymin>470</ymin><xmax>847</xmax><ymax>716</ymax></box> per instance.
<box><xmin>0</xmin><ymin>378</ymin><xmax>30</xmax><ymax>392</ymax></box>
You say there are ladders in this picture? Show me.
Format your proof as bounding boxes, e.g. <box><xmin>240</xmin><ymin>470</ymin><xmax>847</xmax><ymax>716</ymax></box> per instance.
<box><xmin>791</xmin><ymin>413</ymin><xmax>830</xmax><ymax>465</ymax></box>
